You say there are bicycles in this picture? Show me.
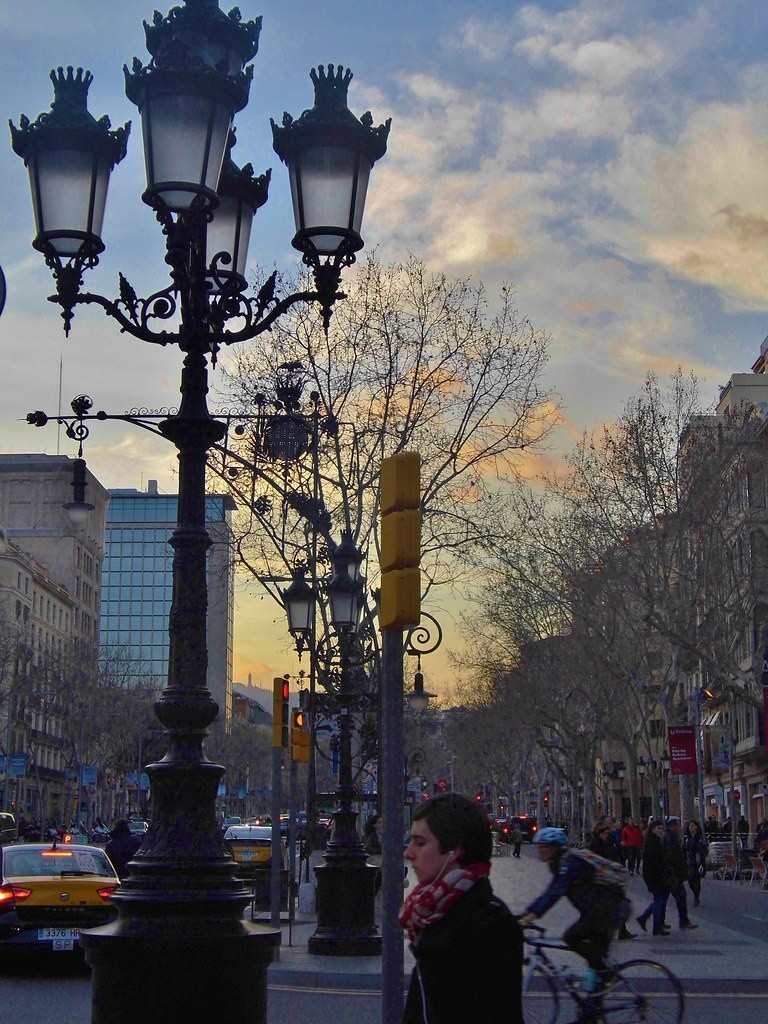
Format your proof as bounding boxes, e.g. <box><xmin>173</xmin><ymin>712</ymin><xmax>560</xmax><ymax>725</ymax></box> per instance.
<box><xmin>514</xmin><ymin>914</ymin><xmax>686</xmax><ymax>1024</ymax></box>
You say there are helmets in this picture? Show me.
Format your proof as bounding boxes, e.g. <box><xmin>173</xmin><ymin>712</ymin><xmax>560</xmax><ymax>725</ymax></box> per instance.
<box><xmin>531</xmin><ymin>827</ymin><xmax>567</xmax><ymax>846</ymax></box>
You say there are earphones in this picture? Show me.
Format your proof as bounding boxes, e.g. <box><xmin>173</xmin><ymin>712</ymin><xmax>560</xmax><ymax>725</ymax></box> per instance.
<box><xmin>448</xmin><ymin>849</ymin><xmax>455</xmax><ymax>856</ymax></box>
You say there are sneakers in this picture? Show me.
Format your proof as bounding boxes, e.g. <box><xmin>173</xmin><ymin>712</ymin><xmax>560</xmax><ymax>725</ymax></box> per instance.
<box><xmin>590</xmin><ymin>970</ymin><xmax>625</xmax><ymax>997</ymax></box>
<box><xmin>567</xmin><ymin>1012</ymin><xmax>605</xmax><ymax>1024</ymax></box>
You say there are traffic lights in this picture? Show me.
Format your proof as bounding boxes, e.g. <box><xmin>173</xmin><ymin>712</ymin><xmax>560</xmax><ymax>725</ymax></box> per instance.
<box><xmin>290</xmin><ymin>707</ymin><xmax>312</xmax><ymax>762</ymax></box>
<box><xmin>272</xmin><ymin>677</ymin><xmax>290</xmax><ymax>747</ymax></box>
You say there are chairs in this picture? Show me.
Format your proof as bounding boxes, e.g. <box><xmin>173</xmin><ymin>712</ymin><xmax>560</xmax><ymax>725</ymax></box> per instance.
<box><xmin>723</xmin><ymin>854</ymin><xmax>745</xmax><ymax>883</ymax></box>
<box><xmin>750</xmin><ymin>858</ymin><xmax>768</xmax><ymax>888</ymax></box>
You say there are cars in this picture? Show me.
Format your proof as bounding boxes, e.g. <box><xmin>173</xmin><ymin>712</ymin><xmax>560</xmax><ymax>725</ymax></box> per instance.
<box><xmin>127</xmin><ymin>820</ymin><xmax>148</xmax><ymax>840</ymax></box>
<box><xmin>500</xmin><ymin>814</ymin><xmax>538</xmax><ymax>844</ymax></box>
<box><xmin>222</xmin><ymin>807</ymin><xmax>333</xmax><ymax>881</ymax></box>
<box><xmin>488</xmin><ymin>813</ymin><xmax>509</xmax><ymax>827</ymax></box>
<box><xmin>0</xmin><ymin>842</ymin><xmax>122</xmax><ymax>971</ymax></box>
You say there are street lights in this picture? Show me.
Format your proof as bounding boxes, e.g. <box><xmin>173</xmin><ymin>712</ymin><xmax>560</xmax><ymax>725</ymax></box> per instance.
<box><xmin>6</xmin><ymin>1</ymin><xmax>393</xmax><ymax>1024</ymax></box>
<box><xmin>281</xmin><ymin>528</ymin><xmax>384</xmax><ymax>958</ymax></box>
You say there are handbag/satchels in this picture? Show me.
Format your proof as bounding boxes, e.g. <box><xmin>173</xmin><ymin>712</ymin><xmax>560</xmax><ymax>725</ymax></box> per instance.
<box><xmin>365</xmin><ymin>854</ymin><xmax>383</xmax><ymax>868</ymax></box>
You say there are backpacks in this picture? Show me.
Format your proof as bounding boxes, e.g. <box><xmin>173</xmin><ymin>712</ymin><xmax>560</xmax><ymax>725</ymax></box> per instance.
<box><xmin>559</xmin><ymin>847</ymin><xmax>628</xmax><ymax>888</ymax></box>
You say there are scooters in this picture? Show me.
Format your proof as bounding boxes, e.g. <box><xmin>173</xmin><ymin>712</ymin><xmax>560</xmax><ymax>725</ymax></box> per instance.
<box><xmin>24</xmin><ymin>821</ymin><xmax>110</xmax><ymax>843</ymax></box>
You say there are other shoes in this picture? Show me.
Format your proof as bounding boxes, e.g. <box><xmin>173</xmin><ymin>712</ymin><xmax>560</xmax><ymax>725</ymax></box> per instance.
<box><xmin>618</xmin><ymin>931</ymin><xmax>638</xmax><ymax>940</ymax></box>
<box><xmin>653</xmin><ymin>925</ymin><xmax>671</xmax><ymax>936</ymax></box>
<box><xmin>636</xmin><ymin>871</ymin><xmax>640</xmax><ymax>875</ymax></box>
<box><xmin>694</xmin><ymin>899</ymin><xmax>700</xmax><ymax>907</ymax></box>
<box><xmin>663</xmin><ymin>923</ymin><xmax>671</xmax><ymax>929</ymax></box>
<box><xmin>679</xmin><ymin>922</ymin><xmax>698</xmax><ymax>929</ymax></box>
<box><xmin>636</xmin><ymin>916</ymin><xmax>648</xmax><ymax>932</ymax></box>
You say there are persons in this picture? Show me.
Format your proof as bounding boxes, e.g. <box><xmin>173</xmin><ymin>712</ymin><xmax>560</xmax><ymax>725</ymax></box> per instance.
<box><xmin>589</xmin><ymin>816</ymin><xmax>768</xmax><ymax>939</ymax></box>
<box><xmin>512</xmin><ymin>823</ymin><xmax>522</xmax><ymax>858</ymax></box>
<box><xmin>363</xmin><ymin>816</ymin><xmax>385</xmax><ymax>902</ymax></box>
<box><xmin>106</xmin><ymin>819</ymin><xmax>143</xmax><ymax>881</ymax></box>
<box><xmin>402</xmin><ymin>792</ymin><xmax>525</xmax><ymax>1024</ymax></box>
<box><xmin>517</xmin><ymin>827</ymin><xmax>631</xmax><ymax>1024</ymax></box>
